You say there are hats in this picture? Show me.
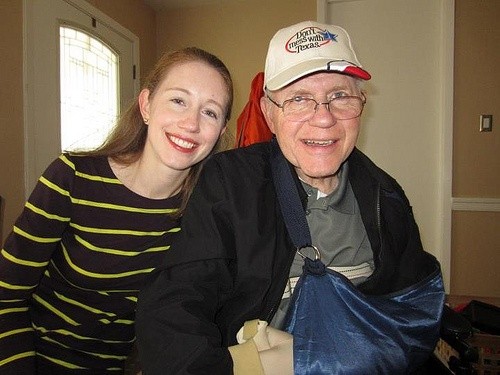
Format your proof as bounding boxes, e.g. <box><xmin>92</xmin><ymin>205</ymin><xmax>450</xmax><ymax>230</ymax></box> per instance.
<box><xmin>262</xmin><ymin>20</ymin><xmax>372</xmax><ymax>92</ymax></box>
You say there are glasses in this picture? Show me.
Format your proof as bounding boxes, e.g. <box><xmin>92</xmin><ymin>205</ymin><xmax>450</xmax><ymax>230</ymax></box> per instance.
<box><xmin>265</xmin><ymin>92</ymin><xmax>367</xmax><ymax>122</ymax></box>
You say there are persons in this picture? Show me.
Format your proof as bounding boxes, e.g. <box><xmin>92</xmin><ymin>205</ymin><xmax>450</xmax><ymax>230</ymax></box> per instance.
<box><xmin>0</xmin><ymin>47</ymin><xmax>233</xmax><ymax>375</ymax></box>
<box><xmin>136</xmin><ymin>20</ymin><xmax>437</xmax><ymax>375</ymax></box>
